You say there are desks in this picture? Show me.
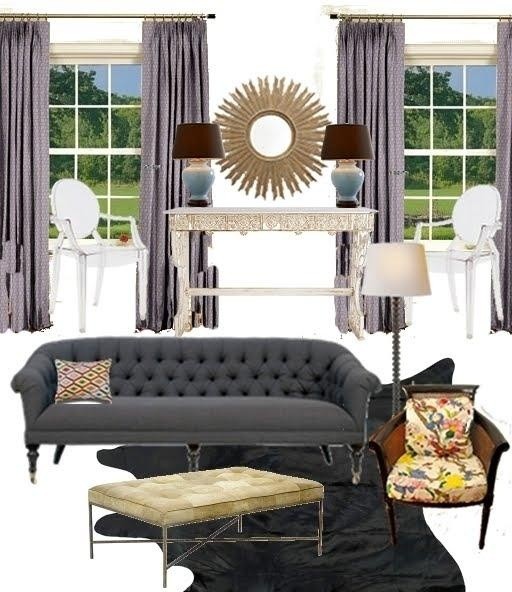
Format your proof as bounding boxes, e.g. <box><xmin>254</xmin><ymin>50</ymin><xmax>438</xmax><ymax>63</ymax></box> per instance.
<box><xmin>162</xmin><ymin>207</ymin><xmax>379</xmax><ymax>339</ymax></box>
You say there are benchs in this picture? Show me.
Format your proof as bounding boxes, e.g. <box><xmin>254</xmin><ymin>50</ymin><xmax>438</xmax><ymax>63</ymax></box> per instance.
<box><xmin>11</xmin><ymin>339</ymin><xmax>382</xmax><ymax>484</ymax></box>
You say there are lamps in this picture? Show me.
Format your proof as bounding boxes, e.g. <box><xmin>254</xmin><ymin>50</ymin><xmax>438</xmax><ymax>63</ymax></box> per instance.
<box><xmin>172</xmin><ymin>123</ymin><xmax>226</xmax><ymax>207</ymax></box>
<box><xmin>361</xmin><ymin>243</ymin><xmax>432</xmax><ymax>418</ymax></box>
<box><xmin>321</xmin><ymin>124</ymin><xmax>375</xmax><ymax>207</ymax></box>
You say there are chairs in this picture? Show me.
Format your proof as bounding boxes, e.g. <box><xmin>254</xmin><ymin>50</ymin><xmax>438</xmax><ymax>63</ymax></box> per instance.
<box><xmin>405</xmin><ymin>184</ymin><xmax>504</xmax><ymax>339</ymax></box>
<box><xmin>48</xmin><ymin>178</ymin><xmax>148</xmax><ymax>333</ymax></box>
<box><xmin>367</xmin><ymin>384</ymin><xmax>510</xmax><ymax>550</ymax></box>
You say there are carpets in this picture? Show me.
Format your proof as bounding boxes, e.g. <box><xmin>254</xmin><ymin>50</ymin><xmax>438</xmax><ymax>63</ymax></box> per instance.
<box><xmin>94</xmin><ymin>445</ymin><xmax>465</xmax><ymax>591</ymax></box>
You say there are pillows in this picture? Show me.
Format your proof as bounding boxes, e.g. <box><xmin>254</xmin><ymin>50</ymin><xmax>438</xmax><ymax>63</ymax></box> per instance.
<box><xmin>54</xmin><ymin>358</ymin><xmax>113</xmax><ymax>405</ymax></box>
<box><xmin>405</xmin><ymin>397</ymin><xmax>474</xmax><ymax>458</ymax></box>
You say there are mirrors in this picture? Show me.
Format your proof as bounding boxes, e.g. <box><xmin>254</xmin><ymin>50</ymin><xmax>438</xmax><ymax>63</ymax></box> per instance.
<box><xmin>245</xmin><ymin>109</ymin><xmax>298</xmax><ymax>162</ymax></box>
